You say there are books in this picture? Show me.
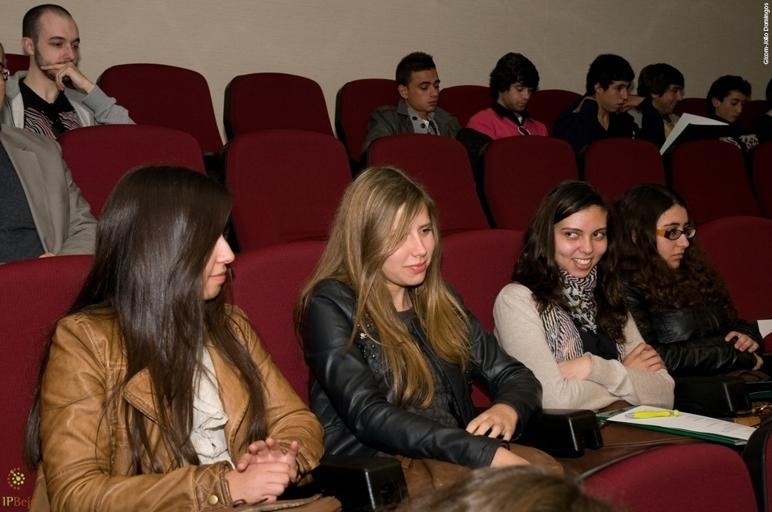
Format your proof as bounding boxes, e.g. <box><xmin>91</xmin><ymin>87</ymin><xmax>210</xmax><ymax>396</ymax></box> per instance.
<box><xmin>660</xmin><ymin>111</ymin><xmax>730</xmax><ymax>158</ymax></box>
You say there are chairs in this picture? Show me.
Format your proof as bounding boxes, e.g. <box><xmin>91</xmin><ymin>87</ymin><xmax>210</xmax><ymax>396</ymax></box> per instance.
<box><xmin>0</xmin><ymin>54</ymin><xmax>771</xmax><ymax>511</ymax></box>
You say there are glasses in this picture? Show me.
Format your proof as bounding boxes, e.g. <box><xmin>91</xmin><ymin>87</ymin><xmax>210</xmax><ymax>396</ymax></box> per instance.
<box><xmin>656</xmin><ymin>228</ymin><xmax>695</xmax><ymax>239</ymax></box>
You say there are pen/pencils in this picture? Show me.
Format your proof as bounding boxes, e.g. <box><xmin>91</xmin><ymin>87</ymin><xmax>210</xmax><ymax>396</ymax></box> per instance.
<box><xmin>625</xmin><ymin>409</ymin><xmax>679</xmax><ymax>419</ymax></box>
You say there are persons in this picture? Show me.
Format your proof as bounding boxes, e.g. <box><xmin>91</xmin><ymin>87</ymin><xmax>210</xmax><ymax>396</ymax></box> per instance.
<box><xmin>8</xmin><ymin>4</ymin><xmax>137</xmax><ymax>139</ymax></box>
<box><xmin>491</xmin><ymin>181</ymin><xmax>756</xmax><ymax>510</ymax></box>
<box><xmin>550</xmin><ymin>54</ymin><xmax>665</xmax><ymax>176</ymax></box>
<box><xmin>637</xmin><ymin>63</ymin><xmax>685</xmax><ymax>173</ymax></box>
<box><xmin>465</xmin><ymin>53</ymin><xmax>548</xmax><ymax>190</ymax></box>
<box><xmin>706</xmin><ymin>75</ymin><xmax>760</xmax><ymax>176</ymax></box>
<box><xmin>359</xmin><ymin>51</ymin><xmax>494</xmax><ymax>170</ymax></box>
<box><xmin>298</xmin><ymin>166</ymin><xmax>565</xmax><ymax>483</ymax></box>
<box><xmin>0</xmin><ymin>40</ymin><xmax>98</xmax><ymax>265</ymax></box>
<box><xmin>616</xmin><ymin>187</ymin><xmax>771</xmax><ymax>417</ymax></box>
<box><xmin>397</xmin><ymin>468</ymin><xmax>608</xmax><ymax>511</ymax></box>
<box><xmin>759</xmin><ymin>79</ymin><xmax>772</xmax><ymax>145</ymax></box>
<box><xmin>30</xmin><ymin>166</ymin><xmax>324</xmax><ymax>511</ymax></box>
